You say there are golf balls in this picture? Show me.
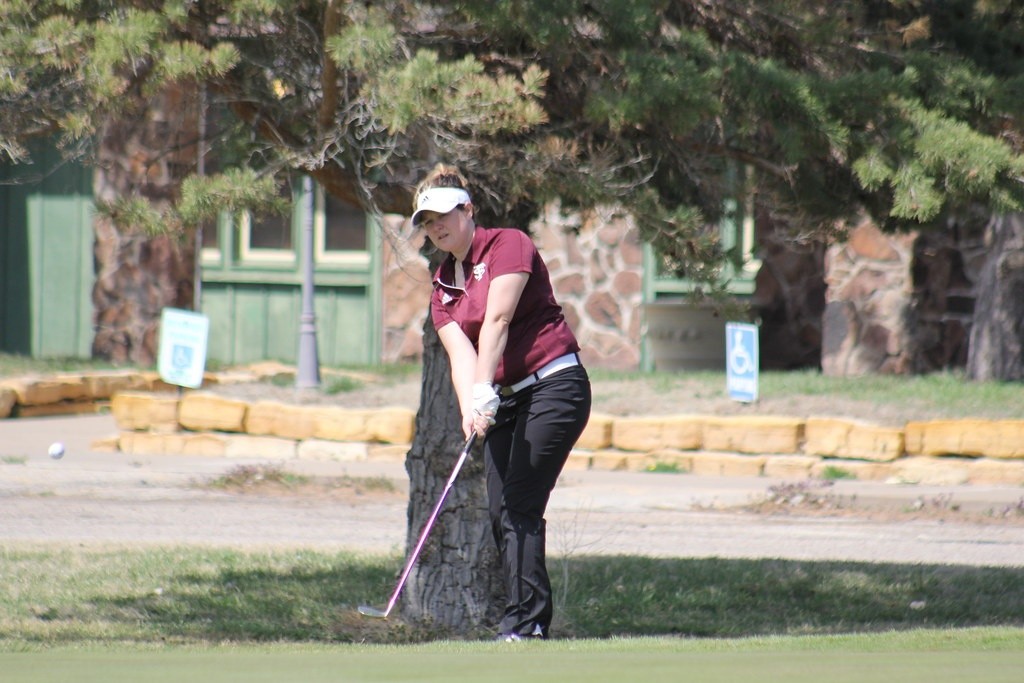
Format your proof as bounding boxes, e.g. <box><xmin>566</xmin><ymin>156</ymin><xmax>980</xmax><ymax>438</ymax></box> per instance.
<box><xmin>48</xmin><ymin>443</ymin><xmax>64</xmax><ymax>459</ymax></box>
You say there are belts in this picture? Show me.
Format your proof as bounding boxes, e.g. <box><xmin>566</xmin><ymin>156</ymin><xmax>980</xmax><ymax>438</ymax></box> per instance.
<box><xmin>502</xmin><ymin>353</ymin><xmax>581</xmax><ymax>397</ymax></box>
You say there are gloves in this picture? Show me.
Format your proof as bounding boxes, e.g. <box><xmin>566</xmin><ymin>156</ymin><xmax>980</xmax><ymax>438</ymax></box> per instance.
<box><xmin>469</xmin><ymin>381</ymin><xmax>502</xmax><ymax>426</ymax></box>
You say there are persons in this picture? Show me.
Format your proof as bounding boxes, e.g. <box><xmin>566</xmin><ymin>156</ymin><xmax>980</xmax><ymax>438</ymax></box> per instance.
<box><xmin>413</xmin><ymin>161</ymin><xmax>592</xmax><ymax>642</ymax></box>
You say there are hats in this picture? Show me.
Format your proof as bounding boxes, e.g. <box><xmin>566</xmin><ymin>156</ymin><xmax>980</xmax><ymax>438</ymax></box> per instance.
<box><xmin>411</xmin><ymin>188</ymin><xmax>473</xmax><ymax>227</ymax></box>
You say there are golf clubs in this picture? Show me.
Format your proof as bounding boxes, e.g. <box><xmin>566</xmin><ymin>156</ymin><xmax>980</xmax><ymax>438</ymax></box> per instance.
<box><xmin>357</xmin><ymin>384</ymin><xmax>503</xmax><ymax>619</ymax></box>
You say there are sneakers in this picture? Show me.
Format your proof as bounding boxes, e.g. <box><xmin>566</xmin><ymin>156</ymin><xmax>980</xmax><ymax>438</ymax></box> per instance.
<box><xmin>492</xmin><ymin>635</ymin><xmax>546</xmax><ymax>644</ymax></box>
